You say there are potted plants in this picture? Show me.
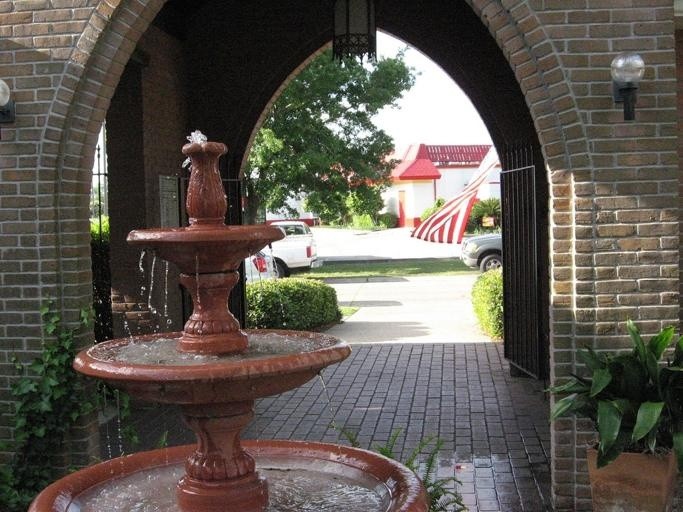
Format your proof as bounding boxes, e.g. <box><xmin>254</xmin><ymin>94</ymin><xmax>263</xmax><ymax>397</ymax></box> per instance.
<box><xmin>541</xmin><ymin>315</ymin><xmax>683</xmax><ymax>512</ymax></box>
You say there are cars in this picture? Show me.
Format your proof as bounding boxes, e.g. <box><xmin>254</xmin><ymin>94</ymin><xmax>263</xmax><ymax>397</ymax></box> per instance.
<box><xmin>245</xmin><ymin>245</ymin><xmax>275</xmax><ymax>282</ymax></box>
<box><xmin>270</xmin><ymin>221</ymin><xmax>312</xmax><ymax>234</ymax></box>
<box><xmin>458</xmin><ymin>235</ymin><xmax>502</xmax><ymax>273</ymax></box>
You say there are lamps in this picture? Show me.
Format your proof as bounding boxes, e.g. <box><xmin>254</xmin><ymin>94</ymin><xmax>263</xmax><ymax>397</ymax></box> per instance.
<box><xmin>0</xmin><ymin>80</ymin><xmax>14</xmax><ymax>123</ymax></box>
<box><xmin>612</xmin><ymin>55</ymin><xmax>645</xmax><ymax>120</ymax></box>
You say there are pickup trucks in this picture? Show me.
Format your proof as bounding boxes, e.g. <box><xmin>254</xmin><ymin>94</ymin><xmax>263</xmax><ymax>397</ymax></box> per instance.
<box><xmin>265</xmin><ymin>226</ymin><xmax>319</xmax><ymax>279</ymax></box>
